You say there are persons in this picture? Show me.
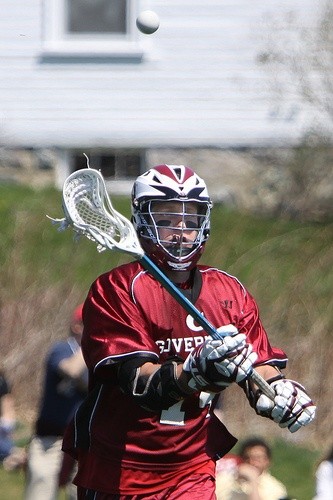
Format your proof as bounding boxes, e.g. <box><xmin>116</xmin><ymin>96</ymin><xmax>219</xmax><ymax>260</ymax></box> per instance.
<box><xmin>61</xmin><ymin>163</ymin><xmax>318</xmax><ymax>500</ymax></box>
<box><xmin>0</xmin><ymin>369</ymin><xmax>27</xmax><ymax>470</ymax></box>
<box><xmin>216</xmin><ymin>437</ymin><xmax>333</xmax><ymax>500</ymax></box>
<box><xmin>23</xmin><ymin>300</ymin><xmax>90</xmax><ymax>500</ymax></box>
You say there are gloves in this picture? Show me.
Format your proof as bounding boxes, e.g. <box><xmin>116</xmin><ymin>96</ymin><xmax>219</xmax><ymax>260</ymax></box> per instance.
<box><xmin>255</xmin><ymin>377</ymin><xmax>317</xmax><ymax>433</ymax></box>
<box><xmin>182</xmin><ymin>324</ymin><xmax>258</xmax><ymax>393</ymax></box>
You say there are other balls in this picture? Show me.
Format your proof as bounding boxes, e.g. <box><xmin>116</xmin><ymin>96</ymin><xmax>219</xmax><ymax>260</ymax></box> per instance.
<box><xmin>136</xmin><ymin>10</ymin><xmax>160</xmax><ymax>34</ymax></box>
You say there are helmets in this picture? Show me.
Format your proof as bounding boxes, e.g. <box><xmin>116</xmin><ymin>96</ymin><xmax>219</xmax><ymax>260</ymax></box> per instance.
<box><xmin>130</xmin><ymin>165</ymin><xmax>213</xmax><ymax>272</ymax></box>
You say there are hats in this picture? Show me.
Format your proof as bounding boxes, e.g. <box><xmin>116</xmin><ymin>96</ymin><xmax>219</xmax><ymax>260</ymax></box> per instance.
<box><xmin>71</xmin><ymin>304</ymin><xmax>85</xmax><ymax>320</ymax></box>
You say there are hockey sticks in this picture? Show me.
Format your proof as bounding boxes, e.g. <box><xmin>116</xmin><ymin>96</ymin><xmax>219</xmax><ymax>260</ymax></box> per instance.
<box><xmin>45</xmin><ymin>153</ymin><xmax>277</xmax><ymax>400</ymax></box>
